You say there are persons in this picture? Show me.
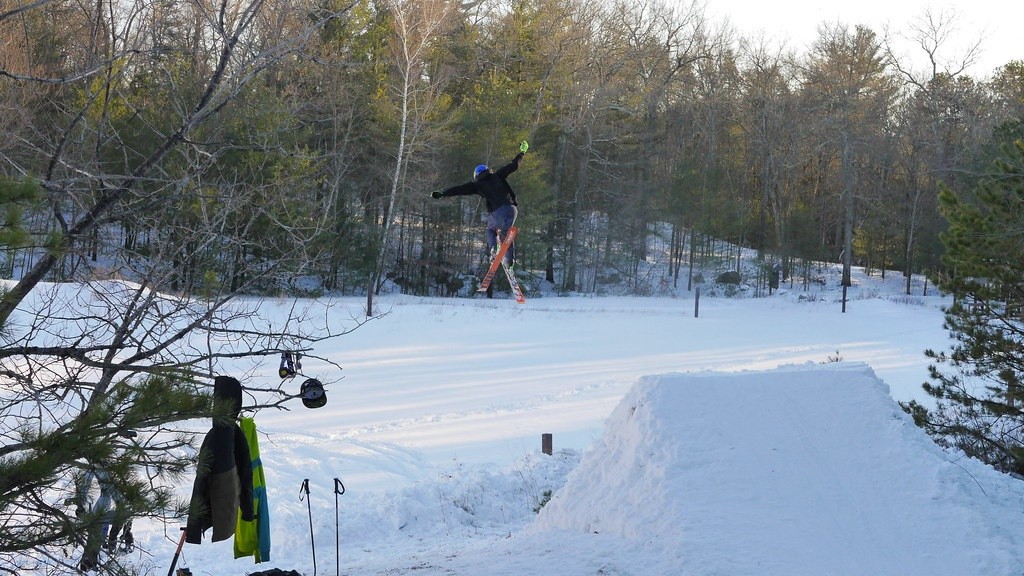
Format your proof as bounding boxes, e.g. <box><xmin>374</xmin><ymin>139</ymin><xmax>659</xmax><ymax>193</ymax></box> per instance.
<box><xmin>429</xmin><ymin>139</ymin><xmax>529</xmax><ymax>270</ymax></box>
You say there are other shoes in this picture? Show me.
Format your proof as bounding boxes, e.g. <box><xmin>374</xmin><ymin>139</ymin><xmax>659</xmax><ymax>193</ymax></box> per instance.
<box><xmin>489</xmin><ymin>246</ymin><xmax>496</xmax><ymax>262</ymax></box>
<box><xmin>502</xmin><ymin>259</ymin><xmax>514</xmax><ymax>268</ymax></box>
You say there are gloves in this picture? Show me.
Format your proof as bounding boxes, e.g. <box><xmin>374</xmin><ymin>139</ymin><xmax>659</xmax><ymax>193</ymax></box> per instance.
<box><xmin>429</xmin><ymin>192</ymin><xmax>442</xmax><ymax>199</ymax></box>
<box><xmin>519</xmin><ymin>140</ymin><xmax>529</xmax><ymax>153</ymax></box>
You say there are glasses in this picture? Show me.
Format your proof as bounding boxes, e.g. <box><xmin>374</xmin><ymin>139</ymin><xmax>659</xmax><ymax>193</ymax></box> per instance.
<box><xmin>279</xmin><ymin>352</ymin><xmax>297</xmax><ymax>378</ymax></box>
<box><xmin>295</xmin><ymin>353</ymin><xmax>302</xmax><ymax>370</ymax></box>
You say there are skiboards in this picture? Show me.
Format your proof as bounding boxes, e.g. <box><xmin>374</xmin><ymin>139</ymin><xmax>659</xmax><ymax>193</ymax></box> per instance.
<box><xmin>476</xmin><ymin>227</ymin><xmax>527</xmax><ymax>304</ymax></box>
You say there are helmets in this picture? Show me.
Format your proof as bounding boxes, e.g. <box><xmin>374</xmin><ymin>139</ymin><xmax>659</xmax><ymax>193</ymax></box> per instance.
<box><xmin>473</xmin><ymin>164</ymin><xmax>493</xmax><ymax>181</ymax></box>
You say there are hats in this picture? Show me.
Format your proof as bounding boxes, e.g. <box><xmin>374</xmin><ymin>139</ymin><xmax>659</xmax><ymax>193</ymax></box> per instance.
<box><xmin>300</xmin><ymin>379</ymin><xmax>327</xmax><ymax>409</ymax></box>
